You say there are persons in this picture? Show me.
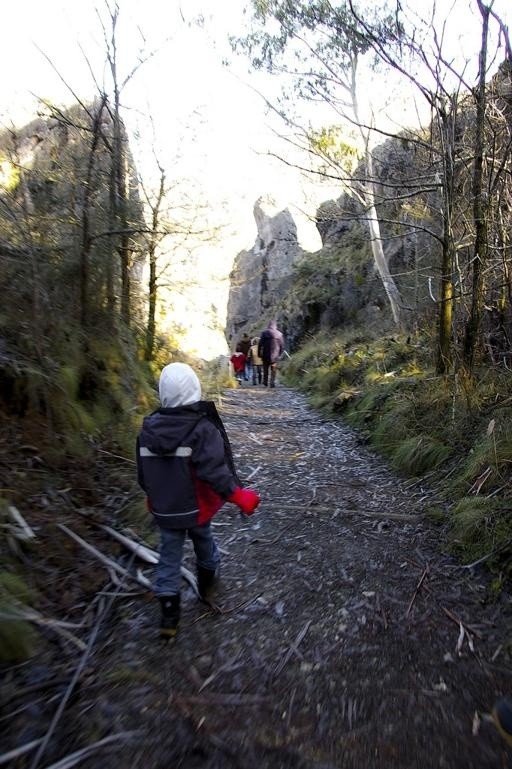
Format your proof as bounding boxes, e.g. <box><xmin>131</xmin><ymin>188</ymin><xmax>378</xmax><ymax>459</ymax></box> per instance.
<box><xmin>231</xmin><ymin>320</ymin><xmax>284</xmax><ymax>388</ymax></box>
<box><xmin>135</xmin><ymin>363</ymin><xmax>260</xmax><ymax>635</ymax></box>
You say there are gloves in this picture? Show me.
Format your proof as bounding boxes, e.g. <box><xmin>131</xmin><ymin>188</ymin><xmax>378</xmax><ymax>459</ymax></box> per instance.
<box><xmin>226</xmin><ymin>485</ymin><xmax>260</xmax><ymax>514</ymax></box>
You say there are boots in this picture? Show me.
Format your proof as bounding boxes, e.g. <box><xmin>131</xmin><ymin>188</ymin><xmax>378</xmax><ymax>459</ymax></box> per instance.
<box><xmin>198</xmin><ymin>565</ymin><xmax>217</xmax><ymax>602</ymax></box>
<box><xmin>159</xmin><ymin>596</ymin><xmax>181</xmax><ymax>635</ymax></box>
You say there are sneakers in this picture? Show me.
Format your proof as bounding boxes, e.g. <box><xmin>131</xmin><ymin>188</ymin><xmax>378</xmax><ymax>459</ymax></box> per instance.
<box><xmin>235</xmin><ymin>375</ymin><xmax>276</xmax><ymax>387</ymax></box>
